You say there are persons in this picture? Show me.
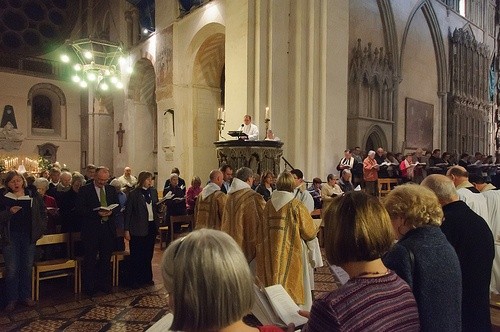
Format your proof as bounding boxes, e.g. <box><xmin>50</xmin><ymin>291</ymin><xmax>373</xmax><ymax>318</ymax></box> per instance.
<box><xmin>262</xmin><ymin>128</ymin><xmax>280</xmax><ymax>140</ymax></box>
<box><xmin>156</xmin><ymin>167</ymin><xmax>357</xmax><ymax>245</ymax></box>
<box><xmin>466</xmin><ymin>166</ymin><xmax>500</xmax><ymax>332</ymax></box>
<box><xmin>261</xmin><ymin>167</ymin><xmax>323</xmax><ymax>311</ymax></box>
<box><xmin>221</xmin><ymin>167</ymin><xmax>269</xmax><ymax>287</ymax></box>
<box><xmin>420</xmin><ymin>172</ymin><xmax>494</xmax><ymax>332</ymax></box>
<box><xmin>239</xmin><ymin>114</ymin><xmax>260</xmax><ymax>141</ymax></box>
<box><xmin>188</xmin><ymin>167</ymin><xmax>230</xmax><ymax>237</ymax></box>
<box><xmin>333</xmin><ymin>144</ymin><xmax>499</xmax><ymax>186</ymax></box>
<box><xmin>0</xmin><ymin>164</ymin><xmax>159</xmax><ymax>294</ymax></box>
<box><xmin>374</xmin><ymin>182</ymin><xmax>462</xmax><ymax>332</ymax></box>
<box><xmin>445</xmin><ymin>164</ymin><xmax>490</xmax><ymax>233</ymax></box>
<box><xmin>283</xmin><ymin>188</ymin><xmax>420</xmax><ymax>332</ymax></box>
<box><xmin>138</xmin><ymin>229</ymin><xmax>284</xmax><ymax>331</ymax></box>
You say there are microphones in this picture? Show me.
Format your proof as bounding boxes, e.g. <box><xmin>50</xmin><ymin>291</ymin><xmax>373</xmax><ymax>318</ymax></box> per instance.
<box><xmin>239</xmin><ymin>124</ymin><xmax>244</xmax><ymax>139</ymax></box>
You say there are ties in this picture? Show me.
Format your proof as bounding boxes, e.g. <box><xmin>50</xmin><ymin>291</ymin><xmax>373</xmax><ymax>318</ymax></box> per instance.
<box><xmin>100</xmin><ymin>188</ymin><xmax>108</xmax><ymax>221</ymax></box>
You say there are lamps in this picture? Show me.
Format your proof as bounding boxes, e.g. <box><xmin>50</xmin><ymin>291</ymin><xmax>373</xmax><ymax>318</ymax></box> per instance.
<box><xmin>60</xmin><ymin>36</ymin><xmax>132</xmax><ymax>90</ymax></box>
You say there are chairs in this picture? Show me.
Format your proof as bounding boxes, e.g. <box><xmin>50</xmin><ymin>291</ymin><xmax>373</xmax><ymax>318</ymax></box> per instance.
<box><xmin>170</xmin><ymin>214</ymin><xmax>194</xmax><ymax>244</ymax></box>
<box><xmin>0</xmin><ymin>230</ymin><xmax>131</xmax><ymax>301</ymax></box>
<box><xmin>157</xmin><ymin>211</ymin><xmax>168</xmax><ymax>250</ymax></box>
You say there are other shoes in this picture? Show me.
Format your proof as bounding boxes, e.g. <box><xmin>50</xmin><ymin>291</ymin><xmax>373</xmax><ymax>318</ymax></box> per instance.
<box><xmin>85</xmin><ymin>285</ymin><xmax>98</xmax><ymax>297</ymax></box>
<box><xmin>97</xmin><ymin>284</ymin><xmax>116</xmax><ymax>295</ymax></box>
<box><xmin>127</xmin><ymin>282</ymin><xmax>140</xmax><ymax>289</ymax></box>
<box><xmin>19</xmin><ymin>298</ymin><xmax>36</xmax><ymax>307</ymax></box>
<box><xmin>4</xmin><ymin>302</ymin><xmax>16</xmax><ymax>311</ymax></box>
<box><xmin>144</xmin><ymin>281</ymin><xmax>154</xmax><ymax>286</ymax></box>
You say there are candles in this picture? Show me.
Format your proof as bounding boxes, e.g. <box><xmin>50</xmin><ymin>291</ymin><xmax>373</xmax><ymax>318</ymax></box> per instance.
<box><xmin>224</xmin><ymin>110</ymin><xmax>226</xmax><ymax>121</ymax></box>
<box><xmin>3</xmin><ymin>157</ymin><xmax>38</xmax><ymax>172</ymax></box>
<box><xmin>218</xmin><ymin>107</ymin><xmax>222</xmax><ymax>119</ymax></box>
<box><xmin>266</xmin><ymin>107</ymin><xmax>269</xmax><ymax>118</ymax></box>
